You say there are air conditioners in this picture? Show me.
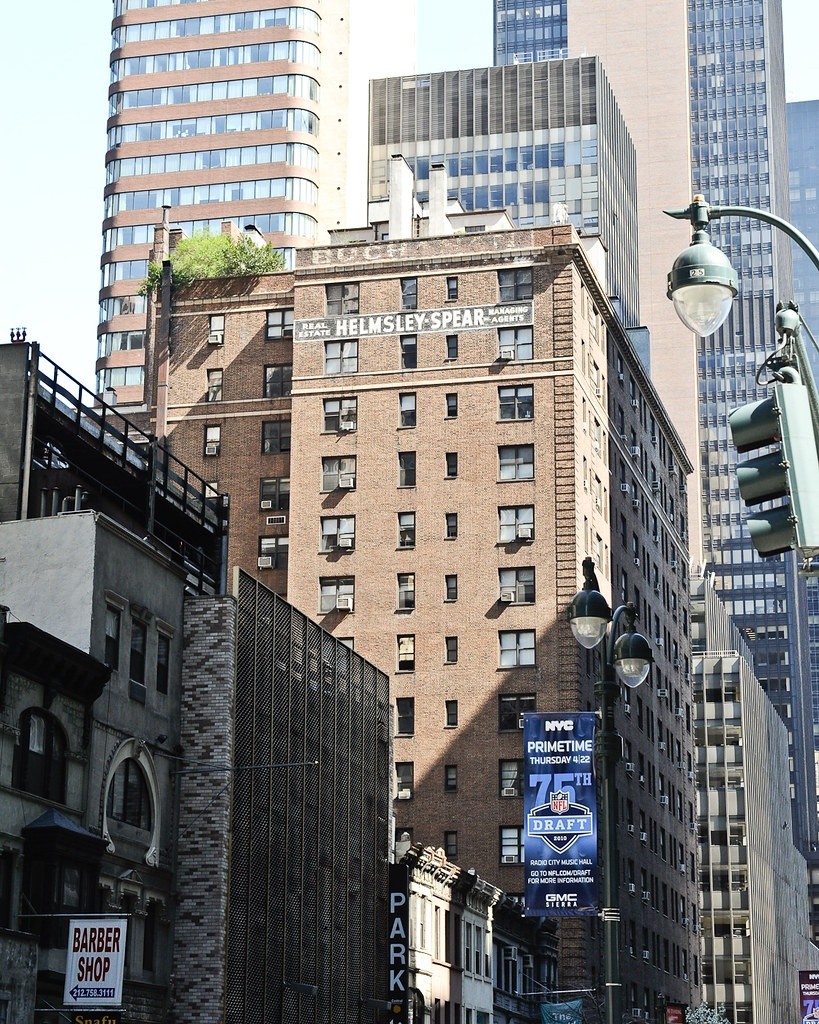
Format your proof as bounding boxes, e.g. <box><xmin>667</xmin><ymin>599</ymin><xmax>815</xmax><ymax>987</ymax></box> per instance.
<box><xmin>582</xmin><ymin>370</ymin><xmax>703</xmax><ymax>985</ymax></box>
<box><xmin>342</xmin><ymin>420</ymin><xmax>354</xmax><ymax>432</ymax></box>
<box><xmin>504</xmin><ymin>856</ymin><xmax>514</xmax><ymax>863</ymax></box>
<box><xmin>504</xmin><ymin>788</ymin><xmax>514</xmax><ymax>796</ymax></box>
<box><xmin>258</xmin><ymin>557</ymin><xmax>273</xmax><ymax>568</ymax></box>
<box><xmin>502</xmin><ymin>349</ymin><xmax>515</xmax><ymax>359</ymax></box>
<box><xmin>632</xmin><ymin>1008</ymin><xmax>641</xmax><ymax>1018</ymax></box>
<box><xmin>518</xmin><ymin>719</ymin><xmax>525</xmax><ymax>729</ymax></box>
<box><xmin>399</xmin><ymin>791</ymin><xmax>412</xmax><ymax>800</ymax></box>
<box><xmin>337</xmin><ymin>597</ymin><xmax>353</xmax><ymax>611</ymax></box>
<box><xmin>262</xmin><ymin>501</ymin><xmax>272</xmax><ymax>508</ymax></box>
<box><xmin>205</xmin><ymin>446</ymin><xmax>217</xmax><ymax>454</ymax></box>
<box><xmin>340</xmin><ymin>539</ymin><xmax>352</xmax><ymax>548</ymax></box>
<box><xmin>209</xmin><ymin>334</ymin><xmax>222</xmax><ymax>344</ymax></box>
<box><xmin>518</xmin><ymin>528</ymin><xmax>531</xmax><ymax>538</ymax></box>
<box><xmin>501</xmin><ymin>591</ymin><xmax>514</xmax><ymax>603</ymax></box>
<box><xmin>644</xmin><ymin>1013</ymin><xmax>649</xmax><ymax>1019</ymax></box>
<box><xmin>339</xmin><ymin>478</ymin><xmax>354</xmax><ymax>489</ymax></box>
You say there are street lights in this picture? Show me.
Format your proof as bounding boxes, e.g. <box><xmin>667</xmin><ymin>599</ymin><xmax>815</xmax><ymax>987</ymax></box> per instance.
<box><xmin>568</xmin><ymin>555</ymin><xmax>656</xmax><ymax>1024</ymax></box>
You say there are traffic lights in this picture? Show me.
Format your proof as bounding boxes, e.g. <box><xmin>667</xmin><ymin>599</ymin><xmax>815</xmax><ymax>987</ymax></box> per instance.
<box><xmin>727</xmin><ymin>382</ymin><xmax>819</xmax><ymax>560</ymax></box>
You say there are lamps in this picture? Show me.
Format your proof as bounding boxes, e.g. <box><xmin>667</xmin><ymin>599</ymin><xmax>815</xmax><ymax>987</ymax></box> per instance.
<box><xmin>157</xmin><ymin>732</ymin><xmax>169</xmax><ymax>743</ymax></box>
